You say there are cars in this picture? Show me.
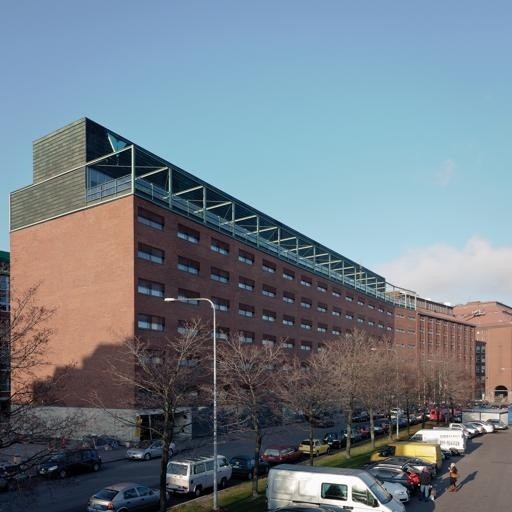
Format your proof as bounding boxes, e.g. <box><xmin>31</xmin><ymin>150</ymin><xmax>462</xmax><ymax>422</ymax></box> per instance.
<box><xmin>36</xmin><ymin>433</ymin><xmax>119</xmax><ymax>480</ymax></box>
<box><xmin>87</xmin><ymin>481</ymin><xmax>170</xmax><ymax>512</ymax></box>
<box><xmin>126</xmin><ymin>439</ymin><xmax>176</xmax><ymax>461</ymax></box>
<box><xmin>230</xmin><ymin>407</ymin><xmax>508</xmax><ymax>512</ymax></box>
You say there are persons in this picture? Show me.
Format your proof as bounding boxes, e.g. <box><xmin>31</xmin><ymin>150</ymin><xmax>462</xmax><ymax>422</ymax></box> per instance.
<box><xmin>448</xmin><ymin>462</ymin><xmax>458</xmax><ymax>493</ymax></box>
<box><xmin>409</xmin><ymin>468</ymin><xmax>421</xmax><ymax>497</ymax></box>
<box><xmin>418</xmin><ymin>468</ymin><xmax>433</xmax><ymax>503</ymax></box>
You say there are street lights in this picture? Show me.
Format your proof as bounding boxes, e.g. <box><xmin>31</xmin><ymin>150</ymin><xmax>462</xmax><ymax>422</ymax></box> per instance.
<box><xmin>371</xmin><ymin>348</ymin><xmax>399</xmax><ymax>437</ymax></box>
<box><xmin>164</xmin><ymin>297</ymin><xmax>220</xmax><ymax>510</ymax></box>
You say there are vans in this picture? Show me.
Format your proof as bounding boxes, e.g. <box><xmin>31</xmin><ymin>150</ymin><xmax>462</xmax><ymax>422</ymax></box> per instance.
<box><xmin>165</xmin><ymin>454</ymin><xmax>232</xmax><ymax>498</ymax></box>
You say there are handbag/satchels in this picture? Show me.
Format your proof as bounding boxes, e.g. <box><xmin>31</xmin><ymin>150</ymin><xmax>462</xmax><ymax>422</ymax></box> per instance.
<box><xmin>450</xmin><ymin>472</ymin><xmax>458</xmax><ymax>479</ymax></box>
